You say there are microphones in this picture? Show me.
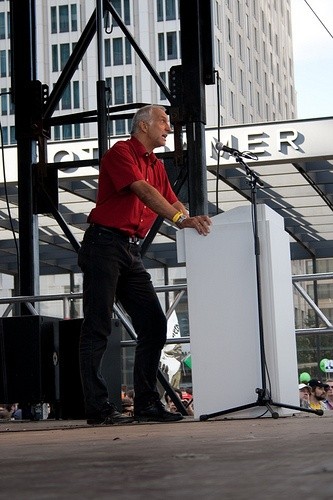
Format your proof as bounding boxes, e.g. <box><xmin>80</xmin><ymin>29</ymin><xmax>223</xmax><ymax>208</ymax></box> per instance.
<box><xmin>215</xmin><ymin>142</ymin><xmax>253</xmax><ymax>160</ymax></box>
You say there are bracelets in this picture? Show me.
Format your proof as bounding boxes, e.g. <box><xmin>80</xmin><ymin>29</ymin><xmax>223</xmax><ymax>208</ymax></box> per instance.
<box><xmin>172</xmin><ymin>212</ymin><xmax>183</xmax><ymax>223</ymax></box>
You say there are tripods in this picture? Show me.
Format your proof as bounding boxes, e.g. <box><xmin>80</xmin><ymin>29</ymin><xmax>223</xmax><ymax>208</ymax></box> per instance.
<box><xmin>198</xmin><ymin>154</ymin><xmax>324</xmax><ymax>422</ymax></box>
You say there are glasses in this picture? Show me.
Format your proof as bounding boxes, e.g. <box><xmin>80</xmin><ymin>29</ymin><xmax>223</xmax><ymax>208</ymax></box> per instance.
<box><xmin>327</xmin><ymin>385</ymin><xmax>333</xmax><ymax>388</ymax></box>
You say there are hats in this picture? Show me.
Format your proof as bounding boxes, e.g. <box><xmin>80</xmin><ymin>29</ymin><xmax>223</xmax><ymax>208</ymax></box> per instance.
<box><xmin>299</xmin><ymin>382</ymin><xmax>312</xmax><ymax>390</ymax></box>
<box><xmin>305</xmin><ymin>378</ymin><xmax>329</xmax><ymax>388</ymax></box>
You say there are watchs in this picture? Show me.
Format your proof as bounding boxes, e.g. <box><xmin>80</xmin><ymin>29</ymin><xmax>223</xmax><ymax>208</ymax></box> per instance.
<box><xmin>176</xmin><ymin>215</ymin><xmax>187</xmax><ymax>227</ymax></box>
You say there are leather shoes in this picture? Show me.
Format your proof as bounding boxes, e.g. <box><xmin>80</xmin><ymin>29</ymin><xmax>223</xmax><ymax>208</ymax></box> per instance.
<box><xmin>87</xmin><ymin>406</ymin><xmax>135</xmax><ymax>425</ymax></box>
<box><xmin>133</xmin><ymin>401</ymin><xmax>182</xmax><ymax>422</ymax></box>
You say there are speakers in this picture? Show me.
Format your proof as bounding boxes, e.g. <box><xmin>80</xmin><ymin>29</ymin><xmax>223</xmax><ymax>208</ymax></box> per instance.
<box><xmin>0</xmin><ymin>315</ymin><xmax>124</xmax><ymax>418</ymax></box>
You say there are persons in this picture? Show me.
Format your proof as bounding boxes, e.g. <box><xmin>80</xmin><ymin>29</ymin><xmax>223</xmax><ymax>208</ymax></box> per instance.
<box><xmin>78</xmin><ymin>105</ymin><xmax>212</xmax><ymax>426</ymax></box>
<box><xmin>121</xmin><ymin>384</ymin><xmax>194</xmax><ymax>416</ymax></box>
<box><xmin>299</xmin><ymin>379</ymin><xmax>333</xmax><ymax>411</ymax></box>
<box><xmin>0</xmin><ymin>401</ymin><xmax>51</xmax><ymax>420</ymax></box>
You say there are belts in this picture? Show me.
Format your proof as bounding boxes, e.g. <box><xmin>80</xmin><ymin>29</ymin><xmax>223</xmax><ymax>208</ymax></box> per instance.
<box><xmin>89</xmin><ymin>223</ymin><xmax>140</xmax><ymax>246</ymax></box>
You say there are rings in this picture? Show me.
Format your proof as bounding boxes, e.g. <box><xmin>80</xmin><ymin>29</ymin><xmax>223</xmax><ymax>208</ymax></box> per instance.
<box><xmin>197</xmin><ymin>222</ymin><xmax>201</xmax><ymax>226</ymax></box>
